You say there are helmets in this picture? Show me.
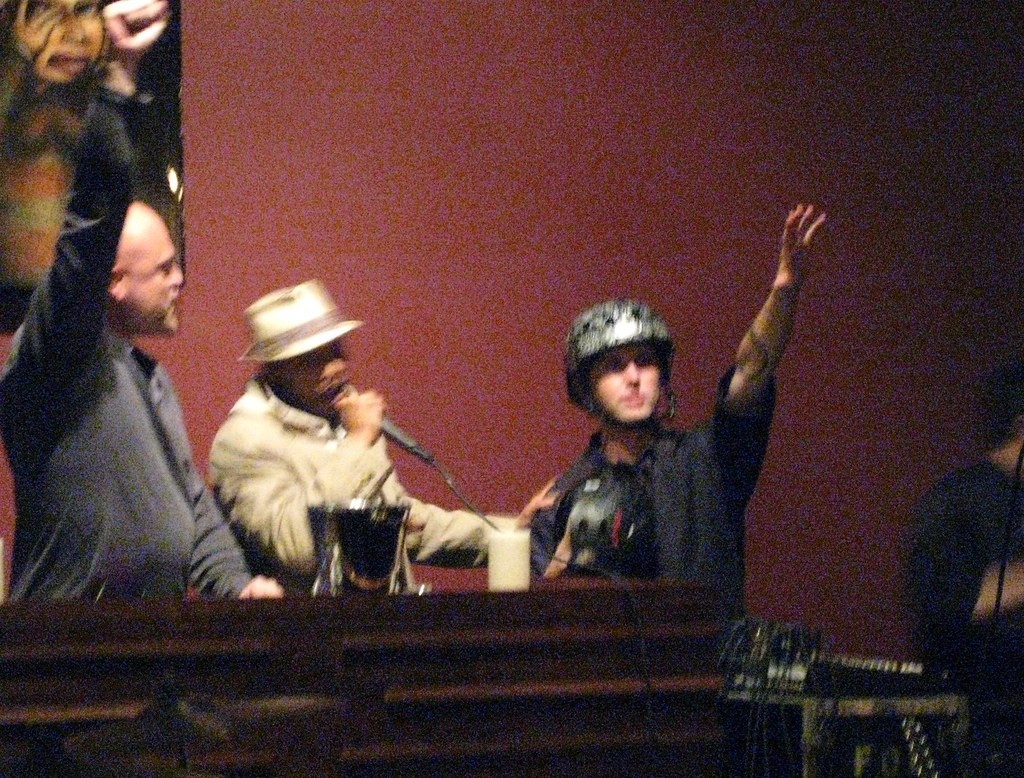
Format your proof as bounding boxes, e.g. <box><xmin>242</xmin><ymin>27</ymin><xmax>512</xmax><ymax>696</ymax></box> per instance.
<box><xmin>565</xmin><ymin>297</ymin><xmax>671</xmax><ymax>402</ymax></box>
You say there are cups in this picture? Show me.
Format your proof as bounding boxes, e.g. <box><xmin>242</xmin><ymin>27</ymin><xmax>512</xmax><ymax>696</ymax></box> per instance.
<box><xmin>488</xmin><ymin>516</ymin><xmax>533</xmax><ymax>593</ymax></box>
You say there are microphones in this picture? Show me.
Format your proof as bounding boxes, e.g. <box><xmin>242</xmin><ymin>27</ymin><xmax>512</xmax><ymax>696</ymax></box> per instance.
<box><xmin>380</xmin><ymin>415</ymin><xmax>435</xmax><ymax>465</ymax></box>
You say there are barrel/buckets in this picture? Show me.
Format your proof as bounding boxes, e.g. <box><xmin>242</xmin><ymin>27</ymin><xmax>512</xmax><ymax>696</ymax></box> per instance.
<box><xmin>307</xmin><ymin>501</ymin><xmax>413</xmax><ymax>597</ymax></box>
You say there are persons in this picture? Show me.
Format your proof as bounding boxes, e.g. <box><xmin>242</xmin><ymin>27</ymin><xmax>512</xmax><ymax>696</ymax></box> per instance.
<box><xmin>530</xmin><ymin>206</ymin><xmax>827</xmax><ymax>633</ymax></box>
<box><xmin>207</xmin><ymin>279</ymin><xmax>557</xmax><ymax>598</ymax></box>
<box><xmin>903</xmin><ymin>366</ymin><xmax>1024</xmax><ymax>778</ymax></box>
<box><xmin>0</xmin><ymin>0</ymin><xmax>286</xmax><ymax>605</ymax></box>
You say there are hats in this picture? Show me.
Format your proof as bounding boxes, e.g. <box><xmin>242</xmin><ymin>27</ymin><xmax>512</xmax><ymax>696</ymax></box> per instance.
<box><xmin>236</xmin><ymin>279</ymin><xmax>364</xmax><ymax>363</ymax></box>
